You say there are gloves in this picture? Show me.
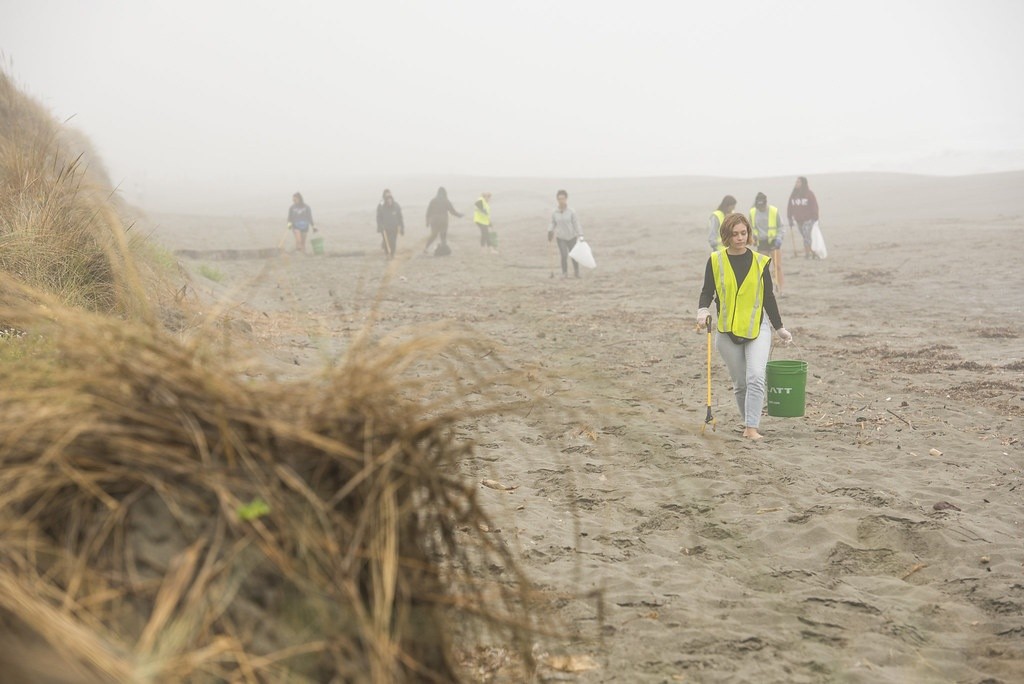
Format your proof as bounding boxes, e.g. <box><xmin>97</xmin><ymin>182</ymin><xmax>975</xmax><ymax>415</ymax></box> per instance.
<box><xmin>789</xmin><ymin>221</ymin><xmax>794</xmax><ymax>226</ymax></box>
<box><xmin>777</xmin><ymin>327</ymin><xmax>792</xmax><ymax>345</ymax></box>
<box><xmin>288</xmin><ymin>222</ymin><xmax>293</xmax><ymax>229</ymax></box>
<box><xmin>696</xmin><ymin>308</ymin><xmax>712</xmax><ymax>329</ymax></box>
<box><xmin>774</xmin><ymin>239</ymin><xmax>781</xmax><ymax>249</ymax></box>
<box><xmin>579</xmin><ymin>236</ymin><xmax>584</xmax><ymax>240</ymax></box>
<box><xmin>311</xmin><ymin>225</ymin><xmax>316</xmax><ymax>232</ymax></box>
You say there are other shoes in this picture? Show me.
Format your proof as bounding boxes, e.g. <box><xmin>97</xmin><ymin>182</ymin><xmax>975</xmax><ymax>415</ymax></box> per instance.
<box><xmin>735</xmin><ymin>420</ymin><xmax>763</xmax><ymax>442</ymax></box>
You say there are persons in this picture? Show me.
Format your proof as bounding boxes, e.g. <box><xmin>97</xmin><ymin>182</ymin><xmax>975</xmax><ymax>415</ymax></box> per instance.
<box><xmin>548</xmin><ymin>190</ymin><xmax>584</xmax><ymax>278</ymax></box>
<box><xmin>708</xmin><ymin>195</ymin><xmax>737</xmax><ymax>253</ymax></box>
<box><xmin>376</xmin><ymin>189</ymin><xmax>405</xmax><ymax>258</ymax></box>
<box><xmin>287</xmin><ymin>192</ymin><xmax>317</xmax><ymax>251</ymax></box>
<box><xmin>786</xmin><ymin>177</ymin><xmax>819</xmax><ymax>260</ymax></box>
<box><xmin>697</xmin><ymin>212</ymin><xmax>792</xmax><ymax>438</ymax></box>
<box><xmin>474</xmin><ymin>192</ymin><xmax>493</xmax><ymax>247</ymax></box>
<box><xmin>424</xmin><ymin>187</ymin><xmax>464</xmax><ymax>248</ymax></box>
<box><xmin>748</xmin><ymin>192</ymin><xmax>784</xmax><ymax>291</ymax></box>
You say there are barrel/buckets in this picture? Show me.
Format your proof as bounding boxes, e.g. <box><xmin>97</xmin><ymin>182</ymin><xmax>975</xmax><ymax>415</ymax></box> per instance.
<box><xmin>310</xmin><ymin>236</ymin><xmax>324</xmax><ymax>255</ymax></box>
<box><xmin>766</xmin><ymin>339</ymin><xmax>808</xmax><ymax>418</ymax></box>
<box><xmin>487</xmin><ymin>232</ymin><xmax>496</xmax><ymax>246</ymax></box>
